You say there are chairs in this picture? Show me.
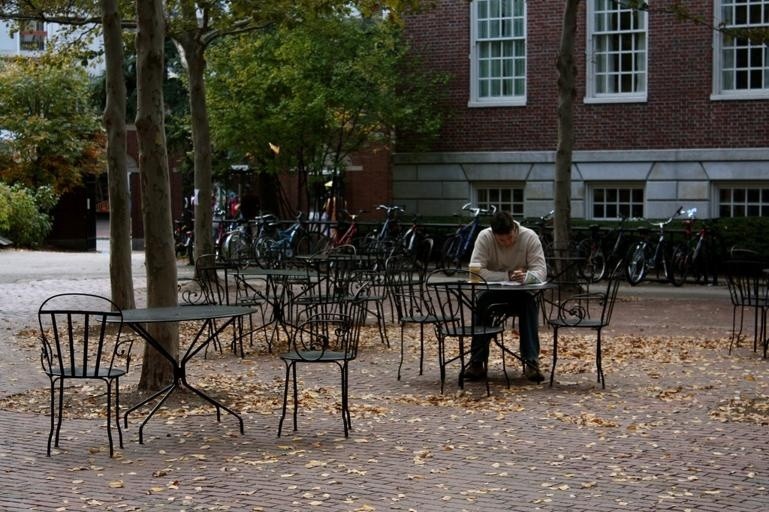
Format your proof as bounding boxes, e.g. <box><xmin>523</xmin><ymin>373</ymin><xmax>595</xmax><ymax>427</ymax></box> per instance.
<box><xmin>275</xmin><ymin>281</ymin><xmax>372</xmax><ymax>437</ymax></box>
<box><xmin>196</xmin><ymin>234</ymin><xmax>626</xmax><ymax>360</ymax></box>
<box><xmin>537</xmin><ymin>258</ymin><xmax>627</xmax><ymax>388</ymax></box>
<box><xmin>39</xmin><ymin>292</ymin><xmax>133</xmax><ymax>458</ymax></box>
<box><xmin>718</xmin><ymin>258</ymin><xmax>768</xmax><ymax>353</ymax></box>
<box><xmin>425</xmin><ymin>269</ymin><xmax>509</xmax><ymax>396</ymax></box>
<box><xmin>385</xmin><ymin>250</ymin><xmax>461</xmax><ymax>380</ymax></box>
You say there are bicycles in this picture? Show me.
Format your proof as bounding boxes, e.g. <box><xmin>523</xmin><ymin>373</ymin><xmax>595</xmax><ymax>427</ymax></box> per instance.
<box><xmin>667</xmin><ymin>216</ymin><xmax>721</xmax><ymax>287</ymax></box>
<box><xmin>624</xmin><ymin>204</ymin><xmax>686</xmax><ymax>288</ymax></box>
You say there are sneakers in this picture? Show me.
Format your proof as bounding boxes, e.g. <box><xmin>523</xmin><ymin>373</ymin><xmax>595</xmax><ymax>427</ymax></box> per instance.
<box><xmin>463</xmin><ymin>366</ymin><xmax>484</xmax><ymax>379</ymax></box>
<box><xmin>525</xmin><ymin>360</ymin><xmax>544</xmax><ymax>381</ymax></box>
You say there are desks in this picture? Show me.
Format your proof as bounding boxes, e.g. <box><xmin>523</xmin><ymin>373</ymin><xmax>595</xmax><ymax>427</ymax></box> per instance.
<box><xmin>424</xmin><ymin>278</ymin><xmax>557</xmax><ymax>386</ymax></box>
<box><xmin>95</xmin><ymin>305</ymin><xmax>258</xmax><ymax>443</ymax></box>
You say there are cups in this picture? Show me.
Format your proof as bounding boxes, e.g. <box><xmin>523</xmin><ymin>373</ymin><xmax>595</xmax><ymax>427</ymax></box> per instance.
<box><xmin>469</xmin><ymin>262</ymin><xmax>482</xmax><ymax>284</ymax></box>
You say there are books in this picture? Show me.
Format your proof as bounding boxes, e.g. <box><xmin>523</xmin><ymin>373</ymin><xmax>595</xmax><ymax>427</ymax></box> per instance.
<box><xmin>466</xmin><ymin>277</ymin><xmax>524</xmax><ymax>287</ymax></box>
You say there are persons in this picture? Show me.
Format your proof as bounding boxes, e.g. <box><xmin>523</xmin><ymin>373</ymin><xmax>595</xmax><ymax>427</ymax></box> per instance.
<box><xmin>459</xmin><ymin>211</ymin><xmax>548</xmax><ymax>384</ymax></box>
